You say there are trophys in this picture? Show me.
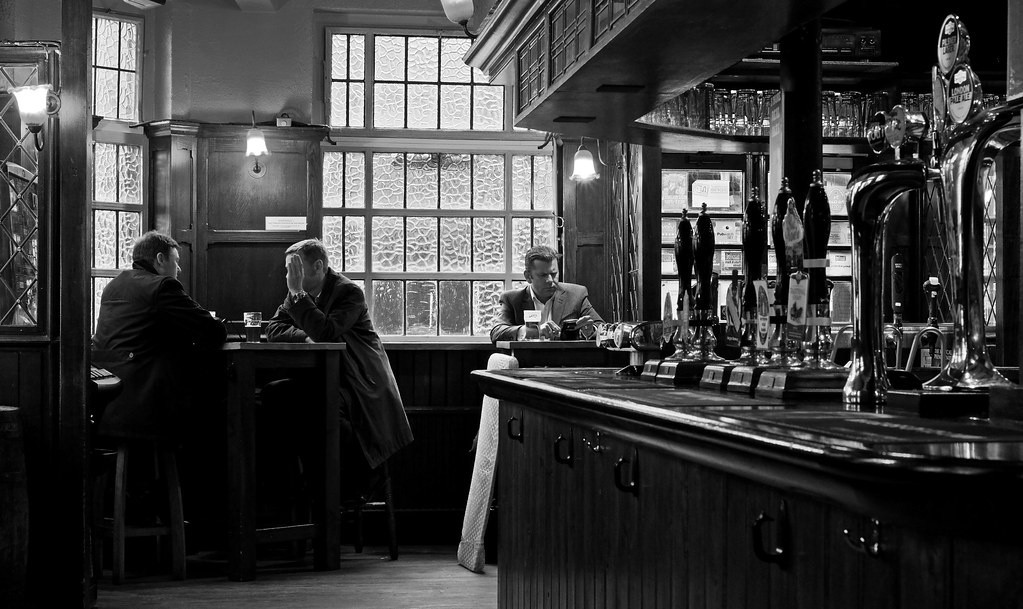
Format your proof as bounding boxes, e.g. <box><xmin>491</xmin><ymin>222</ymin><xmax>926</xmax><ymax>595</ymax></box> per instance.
<box><xmin>641</xmin><ymin>14</ymin><xmax>1023</xmax><ymax>416</ymax></box>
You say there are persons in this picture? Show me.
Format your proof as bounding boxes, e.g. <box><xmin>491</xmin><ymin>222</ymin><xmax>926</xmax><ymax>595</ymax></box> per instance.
<box><xmin>490</xmin><ymin>245</ymin><xmax>604</xmax><ymax>353</ymax></box>
<box><xmin>86</xmin><ymin>232</ymin><xmax>228</xmax><ymax>445</ymax></box>
<box><xmin>264</xmin><ymin>239</ymin><xmax>414</xmax><ymax>507</ymax></box>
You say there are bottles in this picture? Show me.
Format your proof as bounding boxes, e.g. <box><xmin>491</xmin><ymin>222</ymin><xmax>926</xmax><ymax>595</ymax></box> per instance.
<box><xmin>560</xmin><ymin>319</ymin><xmax>580</xmax><ymax>341</ymax></box>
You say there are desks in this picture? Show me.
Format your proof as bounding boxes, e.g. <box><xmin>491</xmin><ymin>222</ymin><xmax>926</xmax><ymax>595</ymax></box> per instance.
<box><xmin>219</xmin><ymin>341</ymin><xmax>345</xmax><ymax>571</ymax></box>
<box><xmin>495</xmin><ymin>337</ymin><xmax>630</xmax><ymax>367</ymax></box>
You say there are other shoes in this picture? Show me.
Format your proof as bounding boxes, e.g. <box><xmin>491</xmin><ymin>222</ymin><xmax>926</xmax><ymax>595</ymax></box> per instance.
<box><xmin>103</xmin><ymin>505</ymin><xmax>119</xmax><ymax>520</ymax></box>
<box><xmin>341</xmin><ymin>493</ymin><xmax>377</xmax><ymax>511</ymax></box>
<box><xmin>146</xmin><ymin>511</ymin><xmax>192</xmax><ymax>529</ymax></box>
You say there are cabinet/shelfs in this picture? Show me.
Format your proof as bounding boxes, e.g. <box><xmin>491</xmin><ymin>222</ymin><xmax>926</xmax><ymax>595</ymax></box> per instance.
<box><xmin>472</xmin><ymin>367</ymin><xmax>1022</xmax><ymax>609</ymax></box>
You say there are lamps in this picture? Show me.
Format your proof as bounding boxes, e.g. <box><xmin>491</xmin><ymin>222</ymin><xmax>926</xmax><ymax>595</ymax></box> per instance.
<box><xmin>441</xmin><ymin>0</ymin><xmax>479</xmax><ymax>39</ymax></box>
<box><xmin>8</xmin><ymin>84</ymin><xmax>52</xmax><ymax>152</ymax></box>
<box><xmin>246</xmin><ymin>110</ymin><xmax>269</xmax><ymax>172</ymax></box>
<box><xmin>570</xmin><ymin>136</ymin><xmax>620</xmax><ymax>180</ymax></box>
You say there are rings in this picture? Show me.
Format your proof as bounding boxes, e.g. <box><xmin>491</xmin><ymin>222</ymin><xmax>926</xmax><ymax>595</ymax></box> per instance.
<box><xmin>585</xmin><ymin>326</ymin><xmax>587</xmax><ymax>329</ymax></box>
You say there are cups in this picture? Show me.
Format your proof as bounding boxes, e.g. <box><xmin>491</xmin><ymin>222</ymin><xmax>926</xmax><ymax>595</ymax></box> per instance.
<box><xmin>636</xmin><ymin>83</ymin><xmax>934</xmax><ymax>137</ymax></box>
<box><xmin>208</xmin><ymin>311</ymin><xmax>215</xmax><ymax>319</ymax></box>
<box><xmin>243</xmin><ymin>312</ymin><xmax>262</xmax><ymax>342</ymax></box>
<box><xmin>523</xmin><ymin>310</ymin><xmax>541</xmax><ymax>339</ymax></box>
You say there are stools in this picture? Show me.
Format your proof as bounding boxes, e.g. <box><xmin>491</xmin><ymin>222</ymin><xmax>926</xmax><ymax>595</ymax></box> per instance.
<box><xmin>90</xmin><ymin>444</ymin><xmax>184</xmax><ymax>583</ymax></box>
<box><xmin>311</xmin><ymin>441</ymin><xmax>402</xmax><ymax>562</ymax></box>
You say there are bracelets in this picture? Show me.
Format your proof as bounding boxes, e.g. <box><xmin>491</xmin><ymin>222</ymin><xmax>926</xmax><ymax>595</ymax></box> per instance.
<box><xmin>292</xmin><ymin>291</ymin><xmax>307</xmax><ymax>302</ymax></box>
